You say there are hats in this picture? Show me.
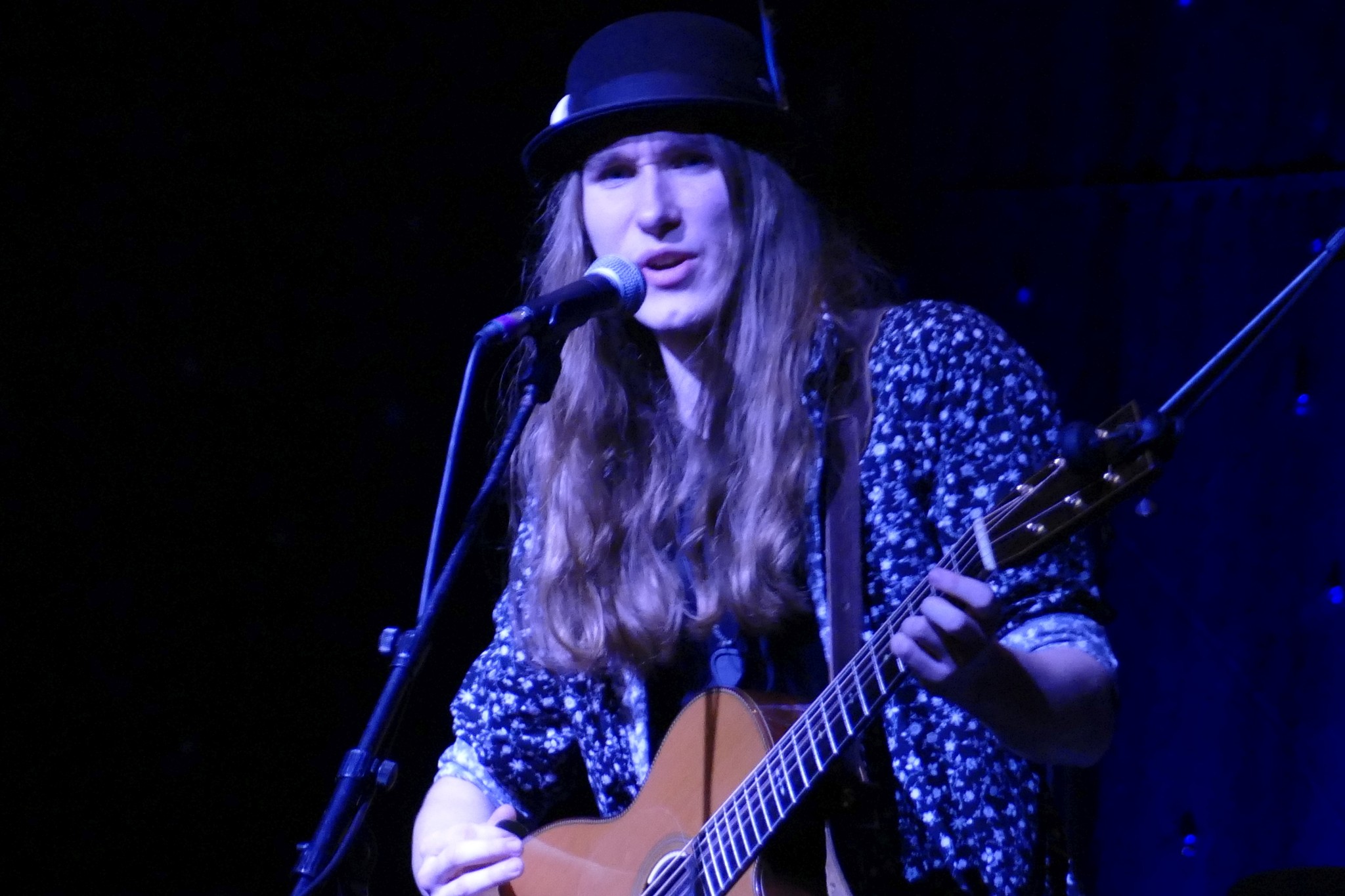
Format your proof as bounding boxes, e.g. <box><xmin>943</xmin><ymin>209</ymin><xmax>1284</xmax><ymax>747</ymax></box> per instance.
<box><xmin>521</xmin><ymin>11</ymin><xmax>780</xmax><ymax>196</ymax></box>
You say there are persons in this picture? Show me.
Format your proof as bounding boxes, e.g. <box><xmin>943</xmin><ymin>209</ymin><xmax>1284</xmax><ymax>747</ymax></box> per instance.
<box><xmin>411</xmin><ymin>13</ymin><xmax>1117</xmax><ymax>896</ymax></box>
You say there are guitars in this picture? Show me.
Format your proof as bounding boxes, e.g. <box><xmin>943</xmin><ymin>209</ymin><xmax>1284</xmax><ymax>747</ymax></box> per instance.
<box><xmin>499</xmin><ymin>404</ymin><xmax>1180</xmax><ymax>896</ymax></box>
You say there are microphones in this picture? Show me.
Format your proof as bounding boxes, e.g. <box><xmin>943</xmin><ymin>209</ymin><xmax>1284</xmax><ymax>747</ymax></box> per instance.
<box><xmin>475</xmin><ymin>250</ymin><xmax>649</xmax><ymax>351</ymax></box>
